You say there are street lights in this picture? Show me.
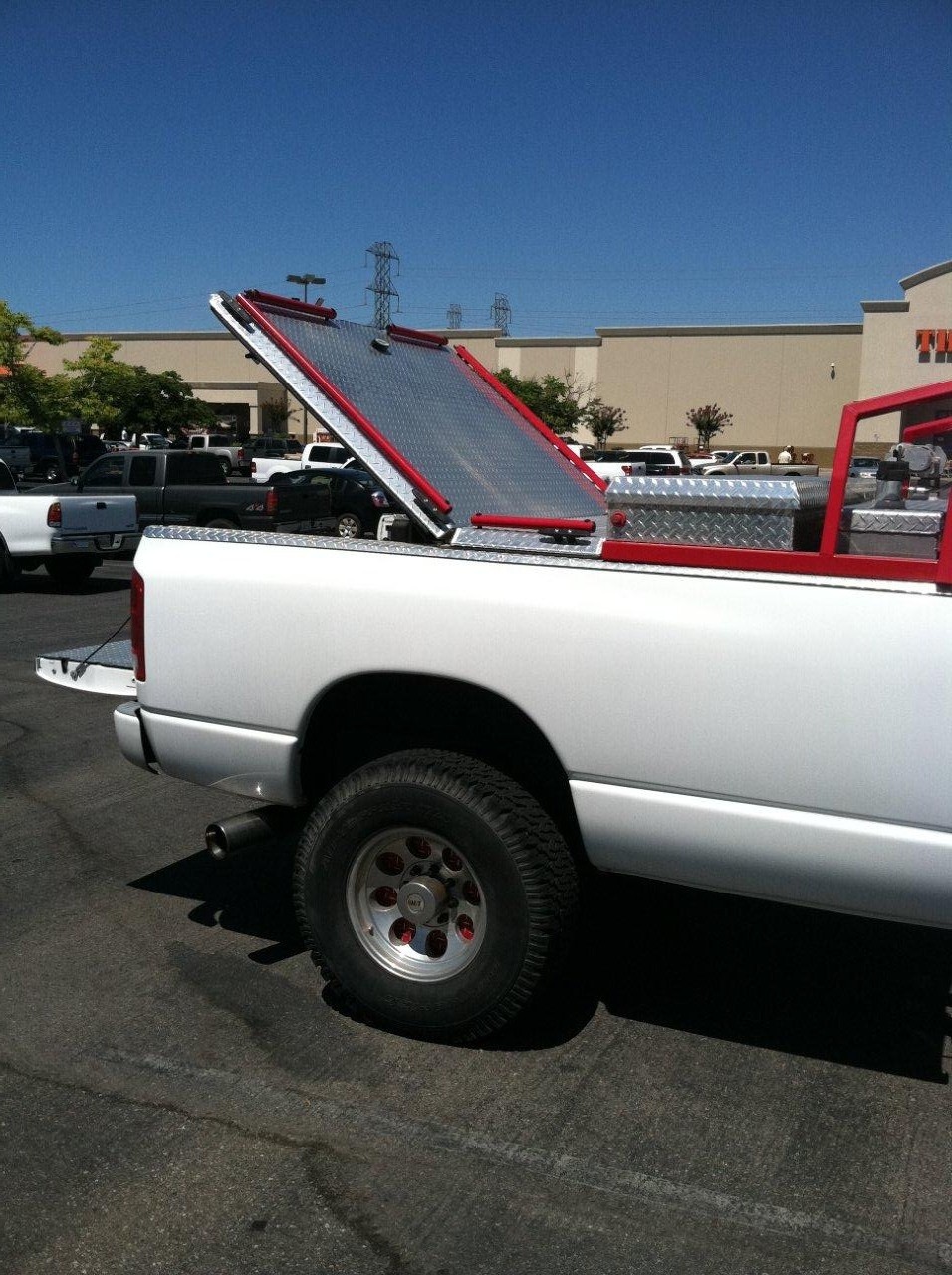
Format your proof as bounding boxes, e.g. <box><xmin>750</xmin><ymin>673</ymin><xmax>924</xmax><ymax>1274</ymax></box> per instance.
<box><xmin>284</xmin><ymin>273</ymin><xmax>326</xmax><ymax>448</ymax></box>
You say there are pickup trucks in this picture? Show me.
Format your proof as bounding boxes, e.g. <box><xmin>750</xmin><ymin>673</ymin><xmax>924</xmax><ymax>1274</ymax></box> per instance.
<box><xmin>25</xmin><ymin>286</ymin><xmax>952</xmax><ymax>1049</ymax></box>
<box><xmin>548</xmin><ymin>436</ymin><xmax>733</xmax><ymax>484</ymax></box>
<box><xmin>697</xmin><ymin>451</ymin><xmax>819</xmax><ymax>478</ymax></box>
<box><xmin>0</xmin><ymin>430</ymin><xmax>368</xmax><ymax>490</ymax></box>
<box><xmin>23</xmin><ymin>449</ymin><xmax>338</xmax><ymax>532</ymax></box>
<box><xmin>0</xmin><ymin>459</ymin><xmax>142</xmax><ymax>596</ymax></box>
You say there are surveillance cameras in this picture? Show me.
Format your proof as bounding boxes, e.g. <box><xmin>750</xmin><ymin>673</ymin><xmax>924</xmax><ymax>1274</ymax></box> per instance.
<box><xmin>831</xmin><ymin>362</ymin><xmax>835</xmax><ymax>367</ymax></box>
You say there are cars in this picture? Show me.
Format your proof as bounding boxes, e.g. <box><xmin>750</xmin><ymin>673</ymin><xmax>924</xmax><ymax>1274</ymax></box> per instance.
<box><xmin>848</xmin><ymin>456</ymin><xmax>882</xmax><ymax>479</ymax></box>
<box><xmin>261</xmin><ymin>468</ymin><xmax>405</xmax><ymax>539</ymax></box>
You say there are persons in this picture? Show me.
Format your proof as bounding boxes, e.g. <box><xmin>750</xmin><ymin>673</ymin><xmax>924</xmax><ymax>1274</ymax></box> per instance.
<box><xmin>777</xmin><ymin>447</ymin><xmax>792</xmax><ymax>464</ymax></box>
<box><xmin>122</xmin><ymin>427</ymin><xmax>128</xmax><ymax>441</ymax></box>
